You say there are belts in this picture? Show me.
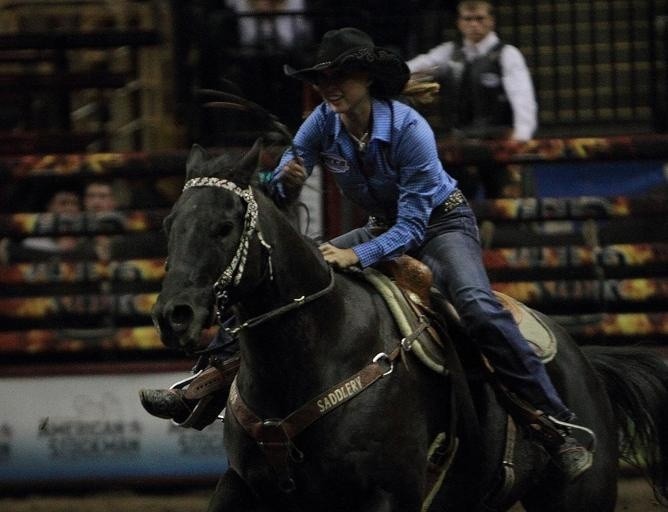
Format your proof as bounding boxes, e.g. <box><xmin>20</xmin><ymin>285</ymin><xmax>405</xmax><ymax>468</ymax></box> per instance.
<box><xmin>364</xmin><ymin>188</ymin><xmax>467</xmax><ymax>230</ymax></box>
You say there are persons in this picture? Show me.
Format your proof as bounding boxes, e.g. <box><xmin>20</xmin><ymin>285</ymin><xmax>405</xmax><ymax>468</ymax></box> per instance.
<box><xmin>0</xmin><ymin>1</ymin><xmax>539</xmax><ymax>373</ymax></box>
<box><xmin>140</xmin><ymin>27</ymin><xmax>597</xmax><ymax>480</ymax></box>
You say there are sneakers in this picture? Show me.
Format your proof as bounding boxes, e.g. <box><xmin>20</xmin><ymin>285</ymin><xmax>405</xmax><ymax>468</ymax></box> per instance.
<box><xmin>137</xmin><ymin>382</ymin><xmax>217</xmax><ymax>434</ymax></box>
<box><xmin>554</xmin><ymin>411</ymin><xmax>594</xmax><ymax>483</ymax></box>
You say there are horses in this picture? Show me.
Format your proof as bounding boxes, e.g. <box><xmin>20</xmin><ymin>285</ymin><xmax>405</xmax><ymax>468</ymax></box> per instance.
<box><xmin>149</xmin><ymin>135</ymin><xmax>668</xmax><ymax>512</ymax></box>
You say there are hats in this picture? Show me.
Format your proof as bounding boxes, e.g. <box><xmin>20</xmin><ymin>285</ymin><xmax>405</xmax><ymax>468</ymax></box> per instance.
<box><xmin>282</xmin><ymin>26</ymin><xmax>412</xmax><ymax>102</ymax></box>
